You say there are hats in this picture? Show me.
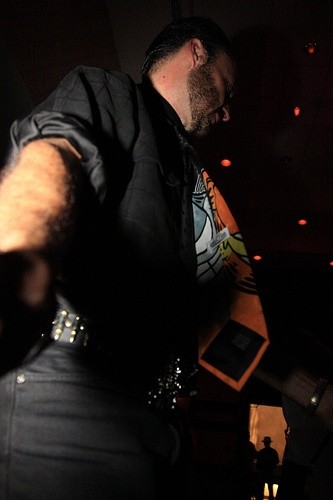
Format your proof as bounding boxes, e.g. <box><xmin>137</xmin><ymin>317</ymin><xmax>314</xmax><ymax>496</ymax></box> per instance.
<box><xmin>262</xmin><ymin>436</ymin><xmax>273</xmax><ymax>441</ymax></box>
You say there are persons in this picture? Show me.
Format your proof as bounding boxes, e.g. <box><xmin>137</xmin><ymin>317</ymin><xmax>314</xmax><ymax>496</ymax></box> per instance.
<box><xmin>248</xmin><ymin>433</ymin><xmax>280</xmax><ymax>499</ymax></box>
<box><xmin>0</xmin><ymin>15</ymin><xmax>238</xmax><ymax>500</ymax></box>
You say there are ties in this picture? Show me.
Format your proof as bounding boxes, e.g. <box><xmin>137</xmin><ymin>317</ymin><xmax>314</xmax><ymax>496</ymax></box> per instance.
<box><xmin>179</xmin><ymin>131</ymin><xmax>270</xmax><ymax>391</ymax></box>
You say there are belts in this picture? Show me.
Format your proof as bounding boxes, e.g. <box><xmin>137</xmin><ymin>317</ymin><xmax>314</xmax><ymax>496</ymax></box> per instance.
<box><xmin>50</xmin><ymin>310</ymin><xmax>107</xmax><ymax>348</ymax></box>
